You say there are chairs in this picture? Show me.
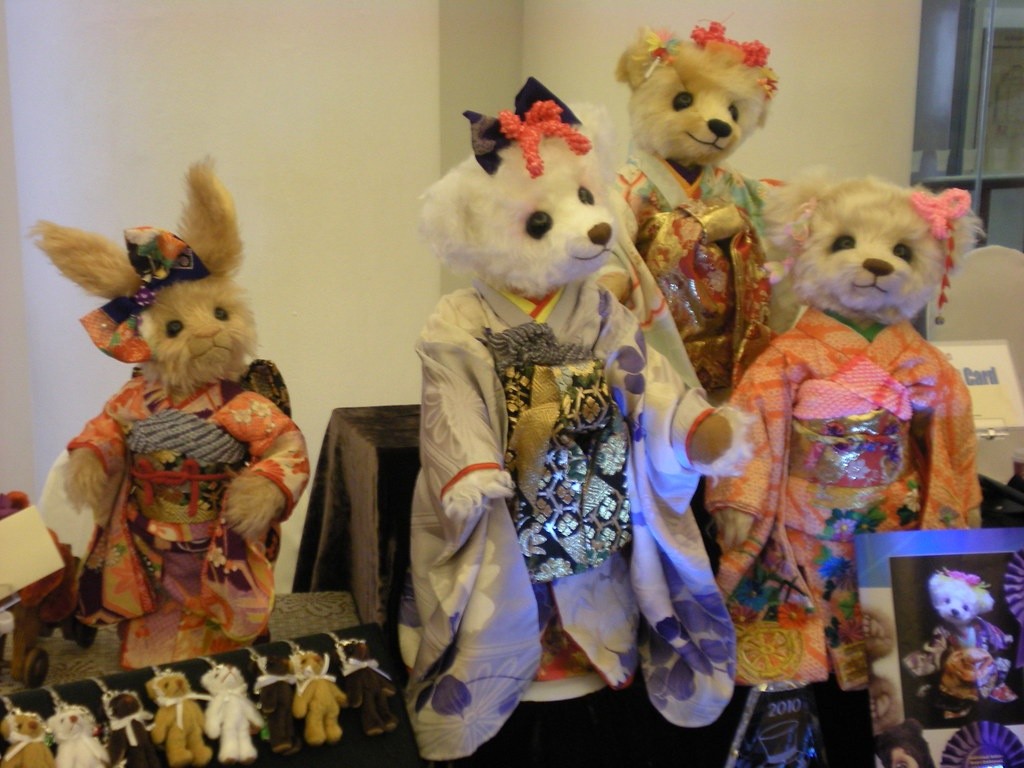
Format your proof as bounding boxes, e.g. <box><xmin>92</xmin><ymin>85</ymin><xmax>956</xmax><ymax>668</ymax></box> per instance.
<box><xmin>927</xmin><ymin>244</ymin><xmax>1024</xmax><ymax>385</ymax></box>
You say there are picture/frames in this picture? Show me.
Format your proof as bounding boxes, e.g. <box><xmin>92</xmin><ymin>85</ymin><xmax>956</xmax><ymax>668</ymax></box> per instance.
<box><xmin>856</xmin><ymin>527</ymin><xmax>1024</xmax><ymax>768</ymax></box>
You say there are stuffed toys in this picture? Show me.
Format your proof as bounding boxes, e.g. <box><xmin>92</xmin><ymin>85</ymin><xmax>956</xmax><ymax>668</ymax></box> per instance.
<box><xmin>247</xmin><ymin>655</ymin><xmax>302</xmax><ymax>755</ymax></box>
<box><xmin>146</xmin><ymin>672</ymin><xmax>215</xmax><ymax>766</ymax></box>
<box><xmin>29</xmin><ymin>154</ymin><xmax>310</xmax><ymax>670</ymax></box>
<box><xmin>289</xmin><ymin>649</ymin><xmax>349</xmax><ymax>745</ymax></box>
<box><xmin>103</xmin><ymin>689</ymin><xmax>163</xmax><ymax>768</ymax></box>
<box><xmin>202</xmin><ymin>664</ymin><xmax>265</xmax><ymax>761</ymax></box>
<box><xmin>340</xmin><ymin>639</ymin><xmax>397</xmax><ymax>733</ymax></box>
<box><xmin>0</xmin><ymin>710</ymin><xmax>56</xmax><ymax>768</ymax></box>
<box><xmin>707</xmin><ymin>168</ymin><xmax>984</xmax><ymax>692</ymax></box>
<box><xmin>612</xmin><ymin>21</ymin><xmax>774</xmax><ymax>415</ymax></box>
<box><xmin>47</xmin><ymin>705</ymin><xmax>110</xmax><ymax>768</ymax></box>
<box><xmin>397</xmin><ymin>76</ymin><xmax>756</xmax><ymax>759</ymax></box>
<box><xmin>904</xmin><ymin>568</ymin><xmax>1017</xmax><ymax>721</ymax></box>
